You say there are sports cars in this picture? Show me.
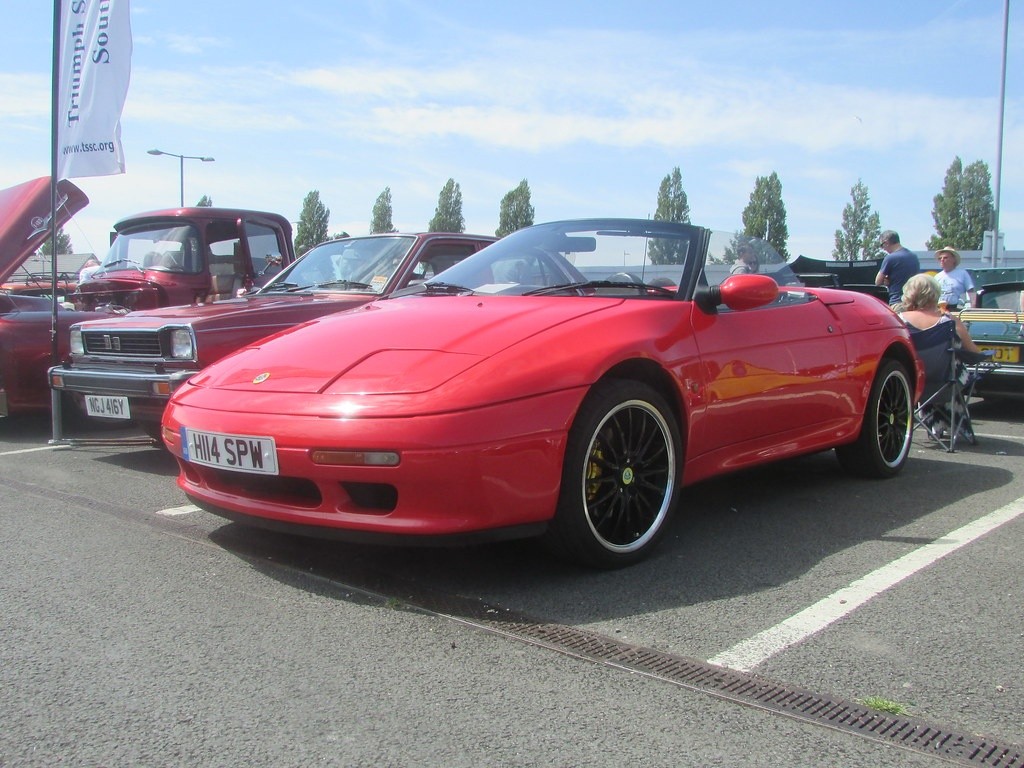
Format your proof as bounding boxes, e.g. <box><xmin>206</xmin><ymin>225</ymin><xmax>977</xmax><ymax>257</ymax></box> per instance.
<box><xmin>155</xmin><ymin>217</ymin><xmax>926</xmax><ymax>571</ymax></box>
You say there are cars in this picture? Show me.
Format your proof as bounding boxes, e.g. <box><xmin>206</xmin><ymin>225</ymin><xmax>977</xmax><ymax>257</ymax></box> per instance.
<box><xmin>1</xmin><ymin>174</ymin><xmax>602</xmax><ymax>449</ymax></box>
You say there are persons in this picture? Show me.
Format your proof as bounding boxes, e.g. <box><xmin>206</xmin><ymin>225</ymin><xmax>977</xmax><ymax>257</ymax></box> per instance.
<box><xmin>875</xmin><ymin>230</ymin><xmax>977</xmax><ymax>316</ymax></box>
<box><xmin>891</xmin><ymin>273</ymin><xmax>982</xmax><ymax>444</ymax></box>
<box><xmin>730</xmin><ymin>243</ymin><xmax>753</xmax><ymax>275</ymax></box>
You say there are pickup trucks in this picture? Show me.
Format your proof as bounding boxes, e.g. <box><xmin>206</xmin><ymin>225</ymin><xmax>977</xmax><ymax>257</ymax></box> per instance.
<box><xmin>947</xmin><ymin>279</ymin><xmax>1024</xmax><ymax>388</ymax></box>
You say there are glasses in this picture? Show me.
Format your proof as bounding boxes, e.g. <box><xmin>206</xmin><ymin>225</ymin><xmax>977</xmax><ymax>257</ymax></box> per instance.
<box><xmin>881</xmin><ymin>242</ymin><xmax>885</xmax><ymax>248</ymax></box>
<box><xmin>937</xmin><ymin>256</ymin><xmax>953</xmax><ymax>261</ymax></box>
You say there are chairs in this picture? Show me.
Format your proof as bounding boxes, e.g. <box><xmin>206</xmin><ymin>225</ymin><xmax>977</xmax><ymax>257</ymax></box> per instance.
<box><xmin>907</xmin><ymin>320</ymin><xmax>996</xmax><ymax>452</ymax></box>
<box><xmin>602</xmin><ymin>273</ymin><xmax>645</xmax><ymax>285</ymax></box>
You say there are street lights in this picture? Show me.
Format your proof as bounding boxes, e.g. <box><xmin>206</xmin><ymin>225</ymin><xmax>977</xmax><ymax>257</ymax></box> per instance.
<box><xmin>147</xmin><ymin>148</ymin><xmax>216</xmax><ymax>208</ymax></box>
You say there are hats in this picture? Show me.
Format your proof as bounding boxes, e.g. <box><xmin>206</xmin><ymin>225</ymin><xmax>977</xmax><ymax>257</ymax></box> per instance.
<box><xmin>934</xmin><ymin>246</ymin><xmax>960</xmax><ymax>266</ymax></box>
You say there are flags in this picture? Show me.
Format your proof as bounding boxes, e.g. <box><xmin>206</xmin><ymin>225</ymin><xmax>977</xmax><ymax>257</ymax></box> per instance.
<box><xmin>54</xmin><ymin>1</ymin><xmax>133</xmax><ymax>179</ymax></box>
